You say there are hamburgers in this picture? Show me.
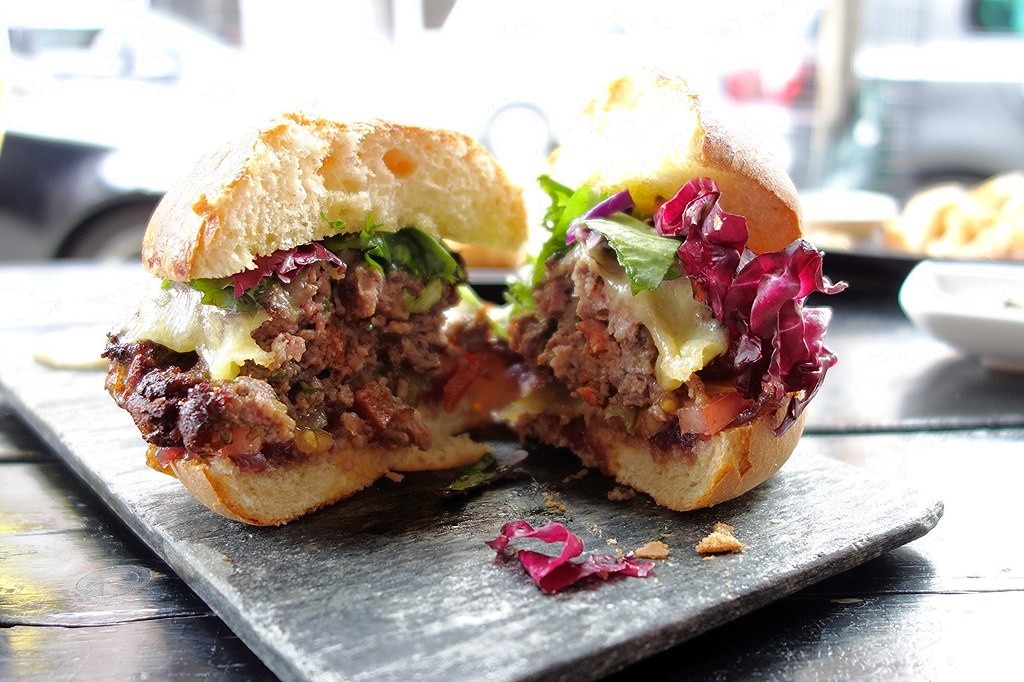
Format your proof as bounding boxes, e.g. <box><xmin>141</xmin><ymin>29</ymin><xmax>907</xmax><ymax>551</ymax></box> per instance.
<box><xmin>105</xmin><ymin>113</ymin><xmax>531</xmax><ymax>524</ymax></box>
<box><xmin>504</xmin><ymin>73</ymin><xmax>848</xmax><ymax>514</ymax></box>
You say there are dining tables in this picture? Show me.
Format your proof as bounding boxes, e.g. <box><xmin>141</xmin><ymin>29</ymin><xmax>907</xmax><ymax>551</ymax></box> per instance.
<box><xmin>0</xmin><ymin>259</ymin><xmax>1023</xmax><ymax>682</ymax></box>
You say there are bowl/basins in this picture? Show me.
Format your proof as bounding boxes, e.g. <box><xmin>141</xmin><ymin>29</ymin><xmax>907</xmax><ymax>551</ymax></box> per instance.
<box><xmin>898</xmin><ymin>260</ymin><xmax>1023</xmax><ymax>374</ymax></box>
<box><xmin>795</xmin><ymin>189</ymin><xmax>896</xmax><ymax>253</ymax></box>
<box><xmin>854</xmin><ymin>33</ymin><xmax>1024</xmax><ymax>175</ymax></box>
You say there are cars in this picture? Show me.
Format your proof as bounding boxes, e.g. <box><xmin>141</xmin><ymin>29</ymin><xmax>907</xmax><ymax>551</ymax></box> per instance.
<box><xmin>0</xmin><ymin>0</ymin><xmax>1024</xmax><ymax>263</ymax></box>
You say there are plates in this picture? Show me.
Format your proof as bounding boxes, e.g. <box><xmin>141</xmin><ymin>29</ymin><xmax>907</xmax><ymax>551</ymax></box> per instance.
<box><xmin>797</xmin><ymin>210</ymin><xmax>1024</xmax><ymax>297</ymax></box>
<box><xmin>0</xmin><ymin>258</ymin><xmax>943</xmax><ymax>682</ymax></box>
